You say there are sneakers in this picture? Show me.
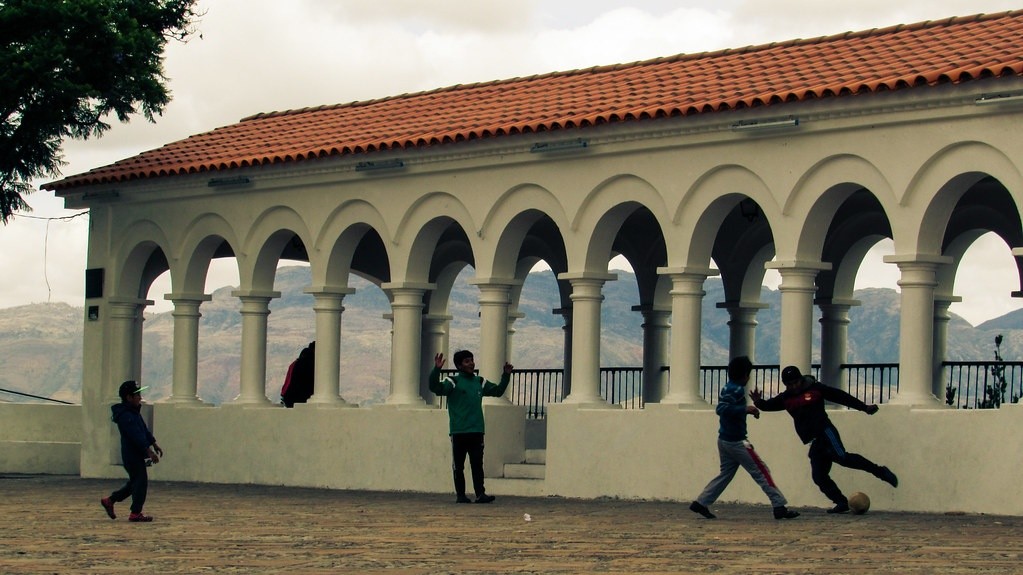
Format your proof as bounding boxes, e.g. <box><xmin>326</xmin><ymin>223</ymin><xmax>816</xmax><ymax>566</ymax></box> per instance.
<box><xmin>101</xmin><ymin>497</ymin><xmax>116</xmax><ymax>520</ymax></box>
<box><xmin>129</xmin><ymin>512</ymin><xmax>153</xmax><ymax>522</ymax></box>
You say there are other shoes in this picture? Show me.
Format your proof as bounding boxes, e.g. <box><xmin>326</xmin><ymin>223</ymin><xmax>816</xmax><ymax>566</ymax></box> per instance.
<box><xmin>773</xmin><ymin>506</ymin><xmax>801</xmax><ymax>519</ymax></box>
<box><xmin>880</xmin><ymin>465</ymin><xmax>898</xmax><ymax>488</ymax></box>
<box><xmin>689</xmin><ymin>500</ymin><xmax>717</xmax><ymax>519</ymax></box>
<box><xmin>827</xmin><ymin>501</ymin><xmax>852</xmax><ymax>514</ymax></box>
<box><xmin>456</xmin><ymin>495</ymin><xmax>472</xmax><ymax>503</ymax></box>
<box><xmin>475</xmin><ymin>492</ymin><xmax>496</xmax><ymax>504</ymax></box>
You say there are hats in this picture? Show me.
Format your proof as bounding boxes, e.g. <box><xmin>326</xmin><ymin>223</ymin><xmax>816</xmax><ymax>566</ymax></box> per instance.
<box><xmin>781</xmin><ymin>366</ymin><xmax>802</xmax><ymax>382</ymax></box>
<box><xmin>118</xmin><ymin>380</ymin><xmax>148</xmax><ymax>400</ymax></box>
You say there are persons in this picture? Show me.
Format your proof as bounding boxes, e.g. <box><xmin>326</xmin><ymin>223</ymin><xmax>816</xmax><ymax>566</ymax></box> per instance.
<box><xmin>689</xmin><ymin>357</ymin><xmax>800</xmax><ymax>519</ymax></box>
<box><xmin>749</xmin><ymin>366</ymin><xmax>899</xmax><ymax>513</ymax></box>
<box><xmin>429</xmin><ymin>350</ymin><xmax>514</xmax><ymax>503</ymax></box>
<box><xmin>100</xmin><ymin>380</ymin><xmax>163</xmax><ymax>522</ymax></box>
<box><xmin>281</xmin><ymin>341</ymin><xmax>315</xmax><ymax>408</ymax></box>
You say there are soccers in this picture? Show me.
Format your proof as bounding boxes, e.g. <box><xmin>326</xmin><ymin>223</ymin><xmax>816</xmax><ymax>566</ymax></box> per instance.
<box><xmin>847</xmin><ymin>491</ymin><xmax>870</xmax><ymax>514</ymax></box>
